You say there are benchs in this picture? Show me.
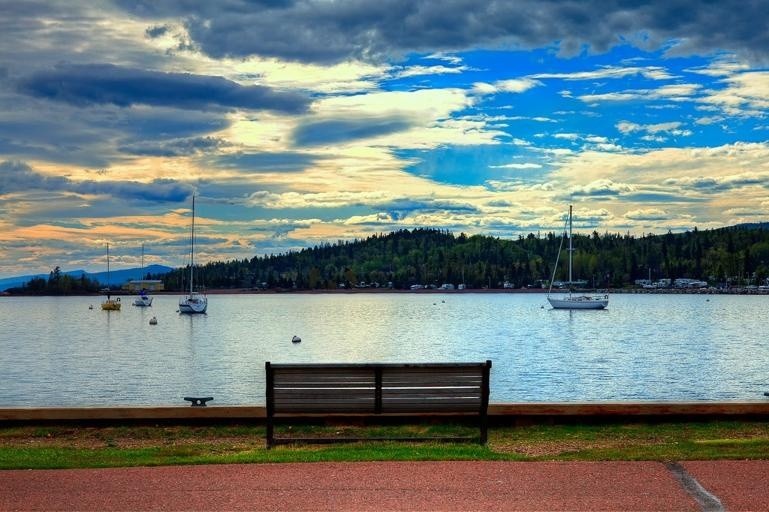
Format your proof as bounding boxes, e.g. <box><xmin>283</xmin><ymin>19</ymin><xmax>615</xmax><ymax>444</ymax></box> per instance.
<box><xmin>265</xmin><ymin>360</ymin><xmax>493</xmax><ymax>446</ymax></box>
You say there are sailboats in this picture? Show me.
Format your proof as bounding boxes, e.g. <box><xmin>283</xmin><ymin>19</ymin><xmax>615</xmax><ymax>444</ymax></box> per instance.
<box><xmin>100</xmin><ymin>242</ymin><xmax>123</xmax><ymax>310</ymax></box>
<box><xmin>131</xmin><ymin>242</ymin><xmax>154</xmax><ymax>307</ymax></box>
<box><xmin>546</xmin><ymin>205</ymin><xmax>610</xmax><ymax>309</ymax></box>
<box><xmin>177</xmin><ymin>194</ymin><xmax>209</xmax><ymax>314</ymax></box>
